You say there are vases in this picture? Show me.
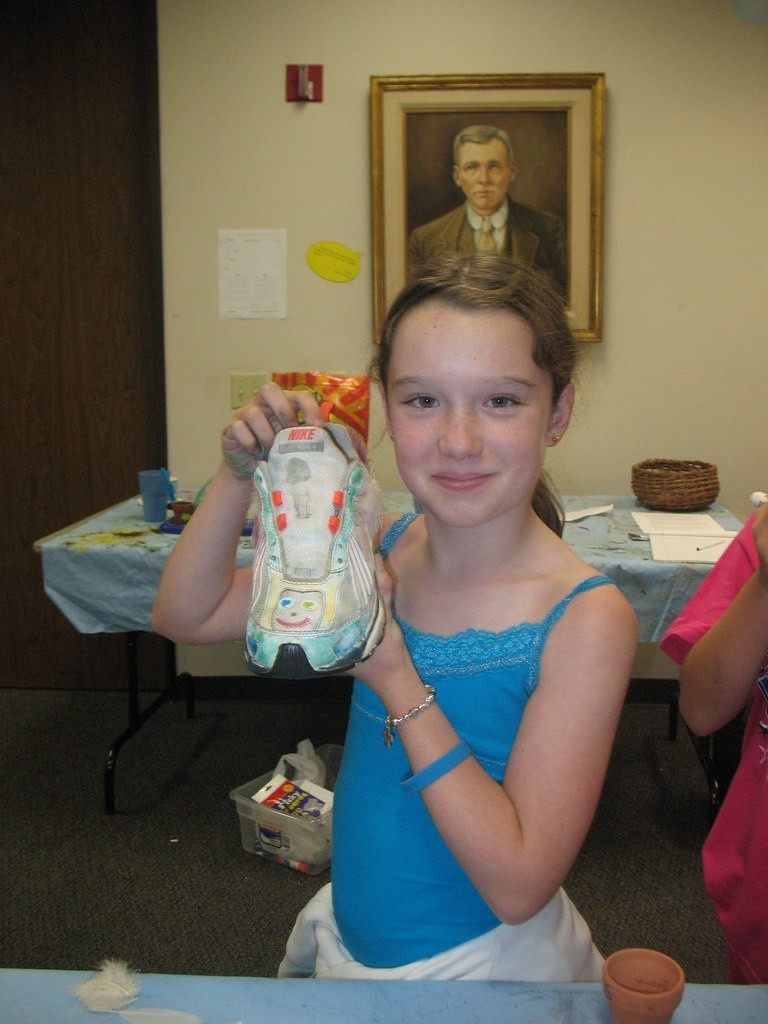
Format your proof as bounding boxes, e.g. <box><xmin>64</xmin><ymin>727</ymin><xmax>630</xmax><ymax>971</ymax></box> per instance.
<box><xmin>171</xmin><ymin>500</ymin><xmax>195</xmax><ymax>524</ymax></box>
<box><xmin>602</xmin><ymin>948</ymin><xmax>686</xmax><ymax>1023</ymax></box>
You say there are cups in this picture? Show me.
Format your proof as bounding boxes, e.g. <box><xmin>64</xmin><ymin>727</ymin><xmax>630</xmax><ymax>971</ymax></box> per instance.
<box><xmin>139</xmin><ymin>470</ymin><xmax>168</xmax><ymax>521</ymax></box>
<box><xmin>172</xmin><ymin>501</ymin><xmax>193</xmax><ymax>523</ymax></box>
<box><xmin>603</xmin><ymin>949</ymin><xmax>684</xmax><ymax>1024</ymax></box>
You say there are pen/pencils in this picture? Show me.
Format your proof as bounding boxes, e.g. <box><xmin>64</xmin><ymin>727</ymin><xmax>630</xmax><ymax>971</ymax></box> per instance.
<box><xmin>697</xmin><ymin>540</ymin><xmax>725</xmax><ymax>551</ymax></box>
<box><xmin>628</xmin><ymin>532</ymin><xmax>650</xmax><ymax>539</ymax></box>
<box><xmin>159</xmin><ymin>468</ymin><xmax>177</xmax><ymax>502</ymax></box>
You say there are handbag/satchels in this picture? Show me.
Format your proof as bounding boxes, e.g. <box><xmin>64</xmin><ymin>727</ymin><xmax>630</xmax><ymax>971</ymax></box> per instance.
<box><xmin>228</xmin><ymin>372</ymin><xmax>371</xmax><ymax>468</ymax></box>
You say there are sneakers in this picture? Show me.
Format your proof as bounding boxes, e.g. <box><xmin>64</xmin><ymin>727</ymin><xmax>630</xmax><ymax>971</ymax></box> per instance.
<box><xmin>250</xmin><ymin>419</ymin><xmax>385</xmax><ymax>679</ymax></box>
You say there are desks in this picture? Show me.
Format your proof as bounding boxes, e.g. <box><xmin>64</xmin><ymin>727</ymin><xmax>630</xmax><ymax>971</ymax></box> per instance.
<box><xmin>33</xmin><ymin>492</ymin><xmax>747</xmax><ymax>817</ymax></box>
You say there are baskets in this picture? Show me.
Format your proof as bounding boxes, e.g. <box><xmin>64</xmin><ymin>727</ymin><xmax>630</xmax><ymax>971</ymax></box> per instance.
<box><xmin>631</xmin><ymin>459</ymin><xmax>720</xmax><ymax>511</ymax></box>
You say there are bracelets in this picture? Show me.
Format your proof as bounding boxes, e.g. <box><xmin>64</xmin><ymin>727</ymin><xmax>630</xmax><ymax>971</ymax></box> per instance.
<box><xmin>382</xmin><ymin>685</ymin><xmax>436</xmax><ymax>747</ymax></box>
<box><xmin>400</xmin><ymin>739</ymin><xmax>473</xmax><ymax>794</ymax></box>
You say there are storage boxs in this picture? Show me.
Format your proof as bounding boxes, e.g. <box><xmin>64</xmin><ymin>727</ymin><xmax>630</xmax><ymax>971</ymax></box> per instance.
<box><xmin>229</xmin><ymin>744</ymin><xmax>343</xmax><ymax>877</ymax></box>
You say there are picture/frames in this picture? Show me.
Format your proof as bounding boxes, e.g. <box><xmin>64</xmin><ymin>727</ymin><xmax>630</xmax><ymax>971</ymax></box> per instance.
<box><xmin>370</xmin><ymin>71</ymin><xmax>603</xmax><ymax>342</ymax></box>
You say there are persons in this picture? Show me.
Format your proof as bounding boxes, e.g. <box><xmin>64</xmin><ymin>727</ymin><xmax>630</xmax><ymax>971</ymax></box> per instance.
<box><xmin>155</xmin><ymin>255</ymin><xmax>638</xmax><ymax>982</ymax></box>
<box><xmin>658</xmin><ymin>499</ymin><xmax>768</xmax><ymax>985</ymax></box>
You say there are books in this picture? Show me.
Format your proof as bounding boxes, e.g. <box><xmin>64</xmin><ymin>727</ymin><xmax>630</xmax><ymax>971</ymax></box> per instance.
<box><xmin>650</xmin><ymin>531</ymin><xmax>737</xmax><ymax>563</ymax></box>
